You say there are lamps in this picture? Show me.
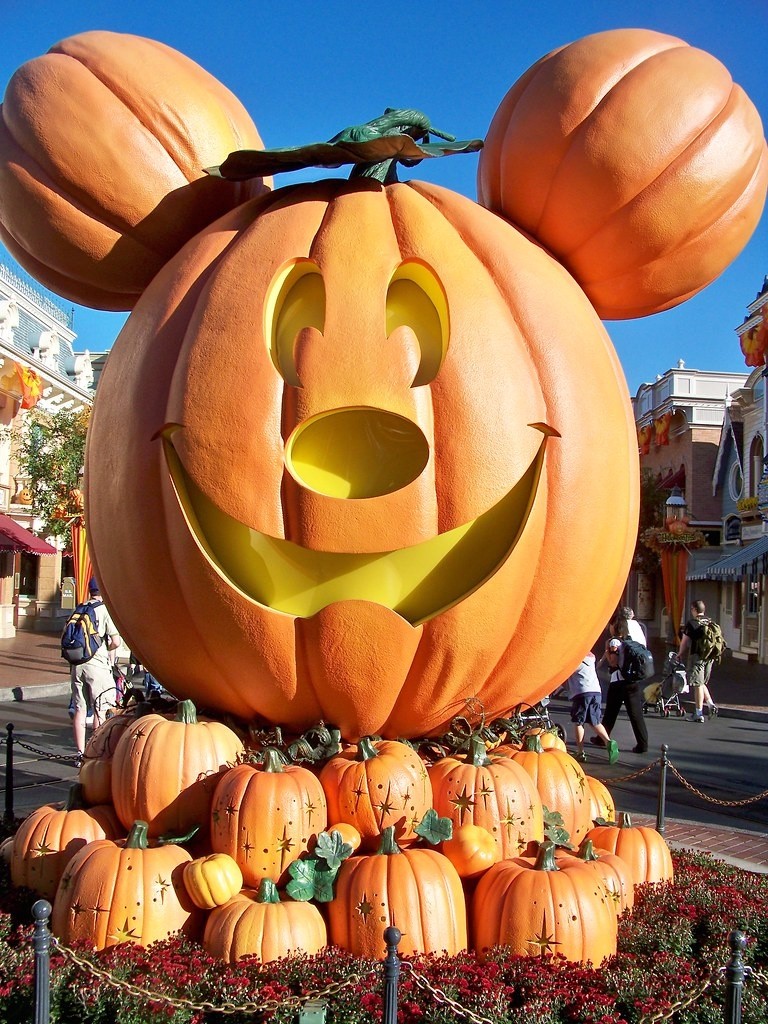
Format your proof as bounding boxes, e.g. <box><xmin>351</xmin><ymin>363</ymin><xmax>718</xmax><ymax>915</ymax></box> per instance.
<box><xmin>665</xmin><ymin>484</ymin><xmax>688</xmax><ymax>520</ymax></box>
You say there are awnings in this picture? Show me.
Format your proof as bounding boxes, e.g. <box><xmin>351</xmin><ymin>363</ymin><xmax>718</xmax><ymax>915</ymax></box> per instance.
<box><xmin>685</xmin><ymin>534</ymin><xmax>768</xmax><ymax>582</ymax></box>
<box><xmin>0</xmin><ymin>513</ymin><xmax>57</xmax><ymax>556</ymax></box>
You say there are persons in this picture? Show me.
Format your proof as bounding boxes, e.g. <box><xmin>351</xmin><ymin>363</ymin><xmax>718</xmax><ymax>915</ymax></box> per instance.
<box><xmin>70</xmin><ymin>576</ymin><xmax>162</xmax><ymax>769</ymax></box>
<box><xmin>556</xmin><ymin>605</ymin><xmax>649</xmax><ymax>763</ymax></box>
<box><xmin>674</xmin><ymin>601</ymin><xmax>718</xmax><ymax>723</ymax></box>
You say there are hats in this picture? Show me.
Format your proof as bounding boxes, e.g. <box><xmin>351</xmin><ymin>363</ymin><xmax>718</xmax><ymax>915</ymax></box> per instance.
<box><xmin>89</xmin><ymin>577</ymin><xmax>100</xmax><ymax>595</ymax></box>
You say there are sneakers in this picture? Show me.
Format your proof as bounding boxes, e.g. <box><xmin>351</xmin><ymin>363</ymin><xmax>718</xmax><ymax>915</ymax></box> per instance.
<box><xmin>685</xmin><ymin>711</ymin><xmax>704</xmax><ymax>723</ymax></box>
<box><xmin>707</xmin><ymin>705</ymin><xmax>718</xmax><ymax>721</ymax></box>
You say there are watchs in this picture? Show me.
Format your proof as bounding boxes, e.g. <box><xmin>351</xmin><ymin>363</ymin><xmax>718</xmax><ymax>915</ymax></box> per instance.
<box><xmin>599</xmin><ymin>659</ymin><xmax>604</xmax><ymax>663</ymax></box>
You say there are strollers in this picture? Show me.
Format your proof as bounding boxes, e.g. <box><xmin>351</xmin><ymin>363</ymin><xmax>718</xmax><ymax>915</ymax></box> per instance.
<box><xmin>641</xmin><ymin>654</ymin><xmax>689</xmax><ymax>718</ymax></box>
<box><xmin>521</xmin><ymin>691</ymin><xmax>567</xmax><ymax>744</ymax></box>
<box><xmin>68</xmin><ymin>663</ymin><xmax>146</xmax><ymax>729</ymax></box>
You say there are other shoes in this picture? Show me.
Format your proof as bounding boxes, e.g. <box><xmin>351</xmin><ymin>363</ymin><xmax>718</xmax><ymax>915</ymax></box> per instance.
<box><xmin>607</xmin><ymin>740</ymin><xmax>619</xmax><ymax>765</ymax></box>
<box><xmin>571</xmin><ymin>752</ymin><xmax>586</xmax><ymax>763</ymax></box>
<box><xmin>633</xmin><ymin>745</ymin><xmax>648</xmax><ymax>753</ymax></box>
<box><xmin>68</xmin><ymin>705</ymin><xmax>74</xmax><ymax>719</ymax></box>
<box><xmin>590</xmin><ymin>737</ymin><xmax>605</xmax><ymax>746</ymax></box>
<box><xmin>75</xmin><ymin>752</ymin><xmax>82</xmax><ymax>767</ymax></box>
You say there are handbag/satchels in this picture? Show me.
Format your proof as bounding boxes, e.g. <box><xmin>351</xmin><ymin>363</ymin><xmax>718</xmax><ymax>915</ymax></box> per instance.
<box><xmin>672</xmin><ymin>670</ymin><xmax>689</xmax><ymax>694</ymax></box>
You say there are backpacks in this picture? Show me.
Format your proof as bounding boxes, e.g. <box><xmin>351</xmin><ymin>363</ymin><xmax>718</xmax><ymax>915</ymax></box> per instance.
<box><xmin>692</xmin><ymin>617</ymin><xmax>727</xmax><ymax>667</ymax></box>
<box><xmin>608</xmin><ymin>635</ymin><xmax>655</xmax><ymax>684</ymax></box>
<box><xmin>60</xmin><ymin>602</ymin><xmax>103</xmax><ymax>666</ymax></box>
